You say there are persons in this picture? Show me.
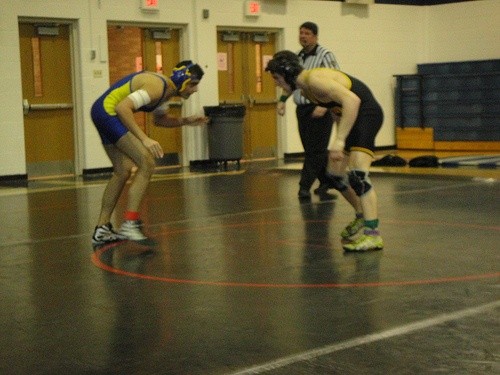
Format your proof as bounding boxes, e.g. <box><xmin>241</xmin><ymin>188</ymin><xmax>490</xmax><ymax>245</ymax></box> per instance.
<box><xmin>276</xmin><ymin>21</ymin><xmax>341</xmax><ymax>200</ymax></box>
<box><xmin>264</xmin><ymin>49</ymin><xmax>384</xmax><ymax>251</ymax></box>
<box><xmin>90</xmin><ymin>61</ymin><xmax>208</xmax><ymax>244</ymax></box>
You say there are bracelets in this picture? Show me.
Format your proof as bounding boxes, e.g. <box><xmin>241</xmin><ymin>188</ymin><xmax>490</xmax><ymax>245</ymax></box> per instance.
<box><xmin>279</xmin><ymin>95</ymin><xmax>288</xmax><ymax>102</ymax></box>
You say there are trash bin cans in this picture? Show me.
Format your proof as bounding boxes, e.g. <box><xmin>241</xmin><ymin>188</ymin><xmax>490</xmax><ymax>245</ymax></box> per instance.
<box><xmin>204</xmin><ymin>104</ymin><xmax>246</xmax><ymax>167</ymax></box>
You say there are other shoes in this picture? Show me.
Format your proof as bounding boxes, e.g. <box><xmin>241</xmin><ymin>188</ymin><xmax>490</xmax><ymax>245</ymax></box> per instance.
<box><xmin>340</xmin><ymin>218</ymin><xmax>363</xmax><ymax>237</ymax></box>
<box><xmin>342</xmin><ymin>227</ymin><xmax>384</xmax><ymax>251</ymax></box>
<box><xmin>117</xmin><ymin>219</ymin><xmax>155</xmax><ymax>246</ymax></box>
<box><xmin>91</xmin><ymin>223</ymin><xmax>121</xmax><ymax>244</ymax></box>
<box><xmin>314</xmin><ymin>183</ymin><xmax>328</xmax><ymax>193</ymax></box>
<box><xmin>298</xmin><ymin>188</ymin><xmax>310</xmax><ymax>199</ymax></box>
